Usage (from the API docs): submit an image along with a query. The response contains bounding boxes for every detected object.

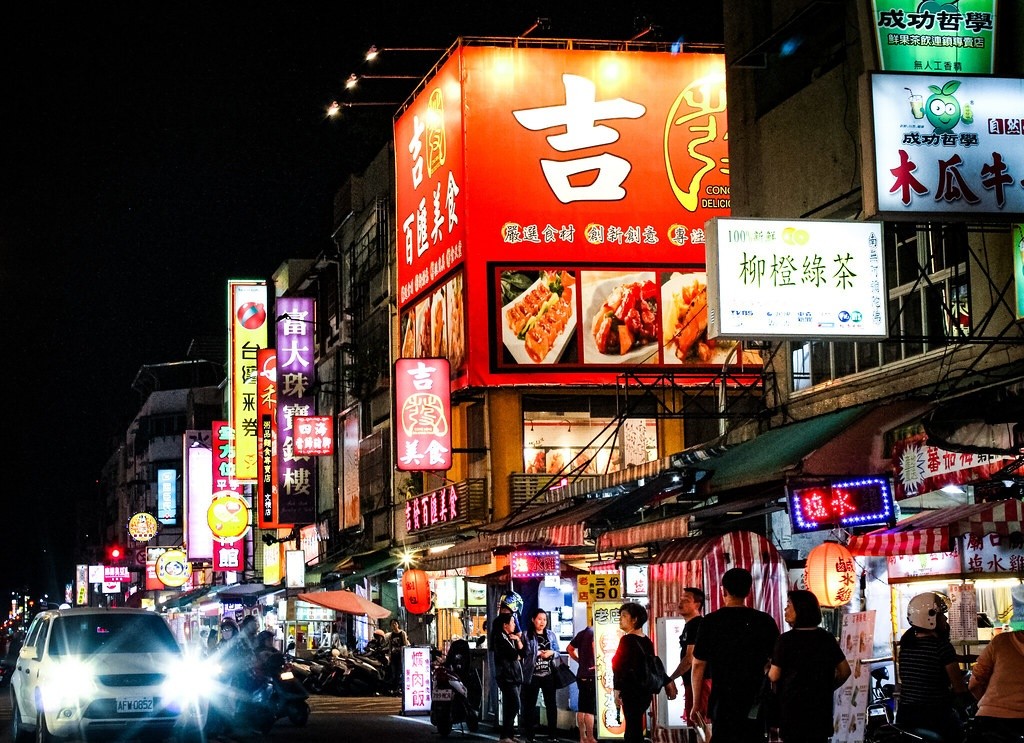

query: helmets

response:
[241,614,257,625]
[906,592,946,629]
[257,631,275,641]
[373,629,385,639]
[499,591,524,616]
[220,617,239,634]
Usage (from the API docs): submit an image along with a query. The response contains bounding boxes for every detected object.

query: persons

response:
[519,609,560,743]
[389,619,411,660]
[566,618,599,743]
[492,614,523,743]
[764,590,852,743]
[690,569,780,743]
[670,587,712,743]
[198,614,279,723]
[968,630,1024,743]
[612,602,678,743]
[896,592,964,732]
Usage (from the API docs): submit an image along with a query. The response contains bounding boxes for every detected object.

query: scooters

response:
[430,639,484,735]
[196,639,401,730]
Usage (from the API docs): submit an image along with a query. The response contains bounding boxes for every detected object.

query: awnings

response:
[156,587,211,611]
[321,547,385,573]
[296,590,391,620]
[597,491,787,555]
[848,499,1024,555]
[345,556,403,582]
[411,497,621,570]
[218,584,285,604]
[690,406,870,487]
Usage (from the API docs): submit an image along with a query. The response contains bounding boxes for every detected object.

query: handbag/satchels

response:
[550,653,576,689]
[618,634,665,694]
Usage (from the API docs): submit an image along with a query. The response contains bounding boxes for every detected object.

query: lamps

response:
[520,16,552,37]
[366,44,447,61]
[328,100,400,115]
[346,73,424,88]
[275,313,316,324]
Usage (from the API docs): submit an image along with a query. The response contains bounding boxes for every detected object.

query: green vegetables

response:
[501,270,563,302]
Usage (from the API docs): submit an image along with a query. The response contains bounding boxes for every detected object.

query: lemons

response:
[520,293,559,333]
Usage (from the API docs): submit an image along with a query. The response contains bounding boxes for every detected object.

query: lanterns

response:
[401,569,431,614]
[804,540,854,609]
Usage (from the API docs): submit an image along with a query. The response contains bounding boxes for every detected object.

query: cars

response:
[8,605,209,743]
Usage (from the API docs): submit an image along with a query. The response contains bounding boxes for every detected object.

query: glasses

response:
[221,629,234,632]
